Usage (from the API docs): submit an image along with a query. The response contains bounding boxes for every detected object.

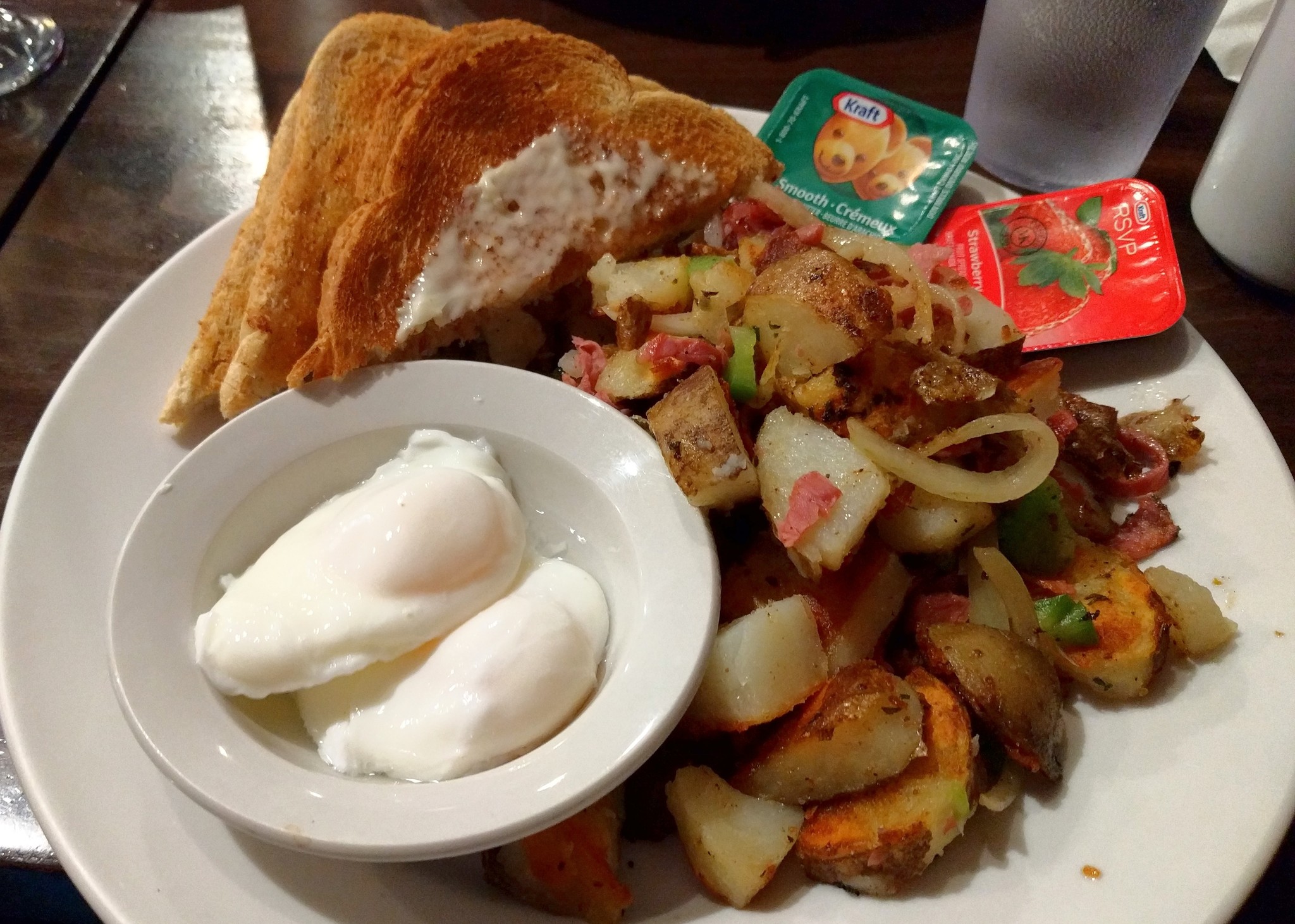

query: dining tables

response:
[3,5,1290,924]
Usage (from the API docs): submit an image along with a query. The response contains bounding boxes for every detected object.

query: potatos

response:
[536,200,1248,905]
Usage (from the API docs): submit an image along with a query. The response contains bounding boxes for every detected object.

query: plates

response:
[99,352,722,870]
[2,102,1293,924]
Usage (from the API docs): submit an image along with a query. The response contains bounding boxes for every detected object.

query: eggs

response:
[178,427,615,791]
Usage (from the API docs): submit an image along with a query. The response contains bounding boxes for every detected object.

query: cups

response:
[1186,0,1295,297]
[961,0,1231,214]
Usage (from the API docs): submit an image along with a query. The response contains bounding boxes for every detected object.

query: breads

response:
[146,13,794,466]
